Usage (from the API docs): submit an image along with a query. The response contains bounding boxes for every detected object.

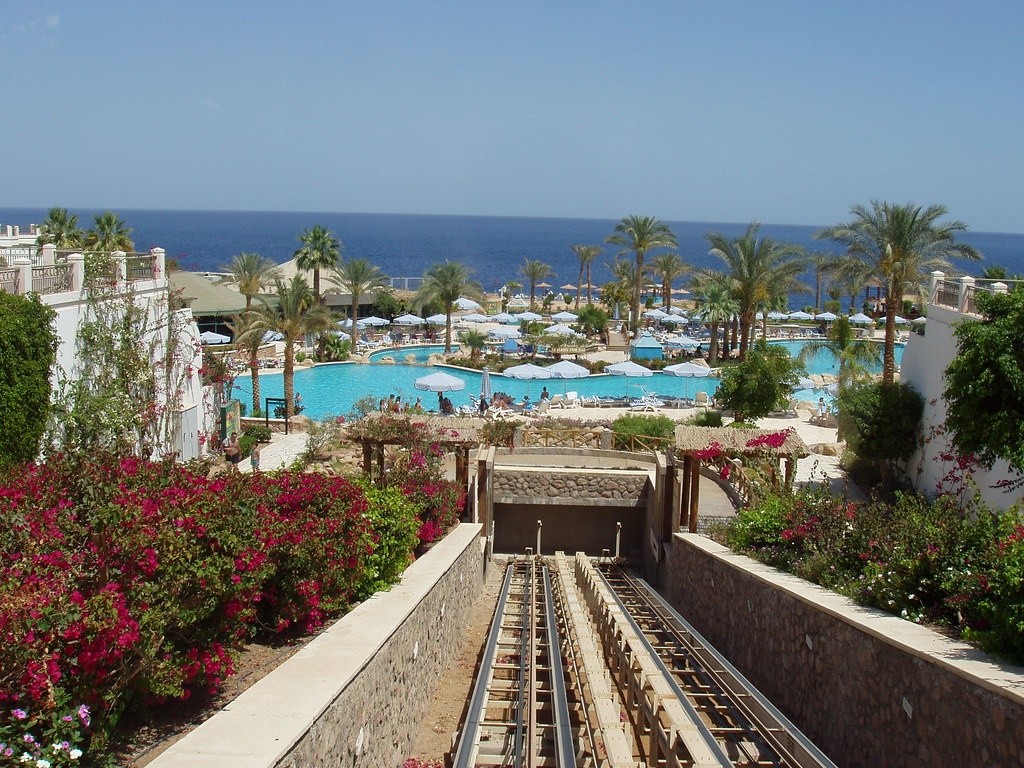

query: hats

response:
[543,387,546,390]
[417,397,422,402]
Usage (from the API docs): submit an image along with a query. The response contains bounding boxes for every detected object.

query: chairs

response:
[228,357,285,372]
[456,391,665,419]
[694,392,709,407]
[809,409,826,421]
[356,338,444,356]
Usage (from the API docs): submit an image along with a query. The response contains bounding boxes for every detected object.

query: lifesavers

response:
[517,346,526,356]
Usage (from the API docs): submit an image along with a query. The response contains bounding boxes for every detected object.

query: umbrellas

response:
[200,330,284,344]
[393,298,583,341]
[544,359,712,406]
[317,316,389,344]
[643,305,926,338]
[414,371,465,392]
[524,282,689,301]
[502,363,553,404]
[665,336,700,357]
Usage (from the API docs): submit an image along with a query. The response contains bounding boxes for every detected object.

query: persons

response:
[250,443,260,472]
[480,343,503,354]
[789,331,794,340]
[541,387,548,399]
[517,343,534,353]
[296,392,301,402]
[221,433,243,473]
[816,396,826,425]
[378,391,535,418]
[711,386,720,408]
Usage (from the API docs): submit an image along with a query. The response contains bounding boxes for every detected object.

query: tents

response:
[631,331,662,360]
